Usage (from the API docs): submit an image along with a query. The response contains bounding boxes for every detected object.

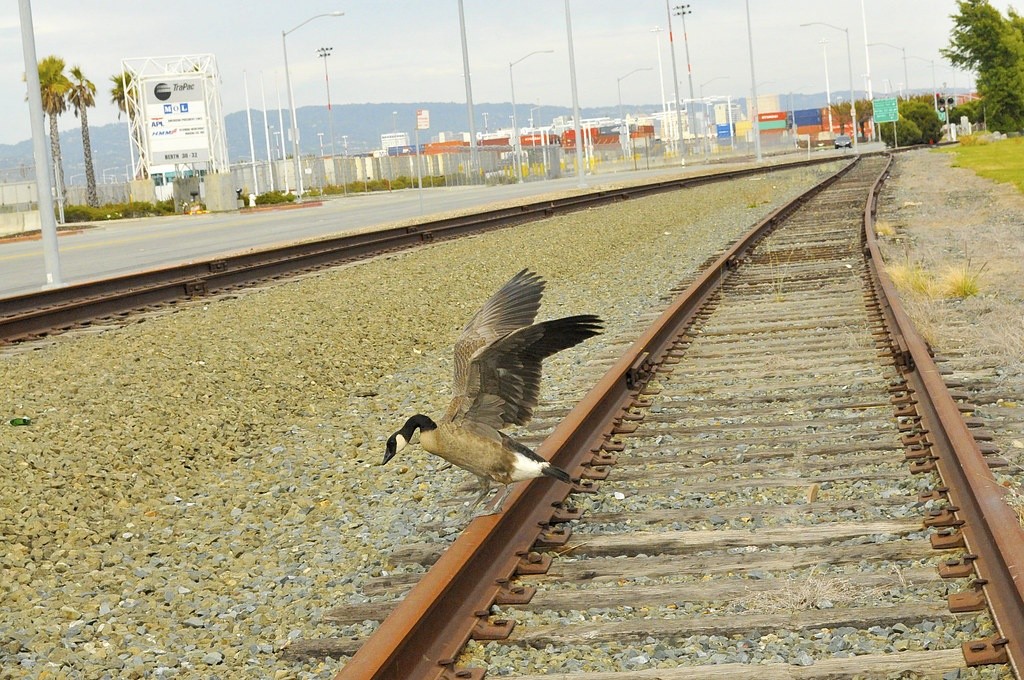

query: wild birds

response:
[382,269,605,482]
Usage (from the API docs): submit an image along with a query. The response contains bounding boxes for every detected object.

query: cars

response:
[833,135,852,149]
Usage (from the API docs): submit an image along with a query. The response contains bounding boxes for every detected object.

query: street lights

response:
[700,74,730,98]
[391,111,400,156]
[481,113,490,134]
[818,37,834,137]
[509,49,555,183]
[342,136,349,157]
[313,46,335,161]
[509,115,516,134]
[283,10,347,201]
[800,22,859,154]
[652,25,672,141]
[672,5,702,156]
[617,67,654,169]
[103,166,120,184]
[902,55,940,116]
[316,132,326,156]
[69,173,84,186]
[273,130,282,157]
[316,133,326,159]
[530,104,545,129]
[126,161,139,183]
[867,43,912,103]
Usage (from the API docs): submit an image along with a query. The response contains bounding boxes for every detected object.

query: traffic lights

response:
[939,98,945,104]
[948,98,954,104]
